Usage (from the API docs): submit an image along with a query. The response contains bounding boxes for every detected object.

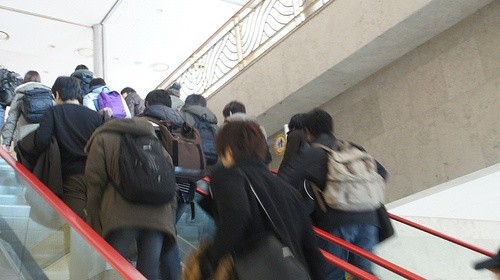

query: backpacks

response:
[76,75,94,103]
[310,139,387,212]
[20,87,54,122]
[98,86,126,118]
[108,131,175,206]
[142,116,206,183]
[193,116,219,164]
[0,69,24,106]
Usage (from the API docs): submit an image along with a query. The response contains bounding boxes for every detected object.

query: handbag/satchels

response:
[232,234,311,280]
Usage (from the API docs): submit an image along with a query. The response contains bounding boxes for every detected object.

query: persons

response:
[121,88,146,116]
[222,101,267,140]
[0,66,24,143]
[282,108,388,280]
[2,71,56,175]
[82,78,133,119]
[278,112,307,174]
[181,95,220,166]
[166,83,184,111]
[71,65,95,94]
[34,77,104,223]
[191,120,332,280]
[82,116,178,280]
[138,90,205,220]
